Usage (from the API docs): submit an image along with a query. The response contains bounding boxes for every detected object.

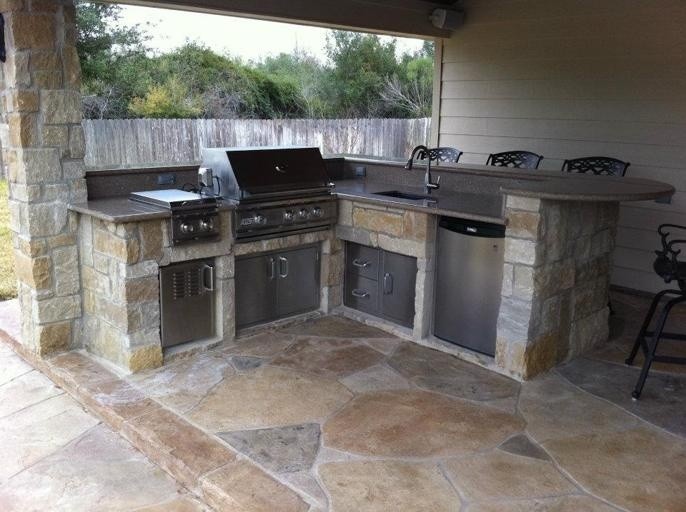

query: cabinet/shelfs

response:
[158,258,217,349]
[381,240,416,328]
[345,244,380,312]
[234,241,322,331]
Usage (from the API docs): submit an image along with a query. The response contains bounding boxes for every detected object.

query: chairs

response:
[485,151,544,170]
[417,146,463,166]
[561,156,631,177]
[629,223,685,398]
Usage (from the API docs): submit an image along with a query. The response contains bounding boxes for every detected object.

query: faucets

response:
[405,145,441,194]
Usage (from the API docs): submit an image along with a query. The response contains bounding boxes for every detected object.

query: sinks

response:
[371,190,434,201]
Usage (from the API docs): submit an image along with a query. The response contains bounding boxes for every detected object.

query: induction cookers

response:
[126,188,221,245]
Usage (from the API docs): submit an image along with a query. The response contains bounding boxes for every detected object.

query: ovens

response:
[433,216,506,357]
